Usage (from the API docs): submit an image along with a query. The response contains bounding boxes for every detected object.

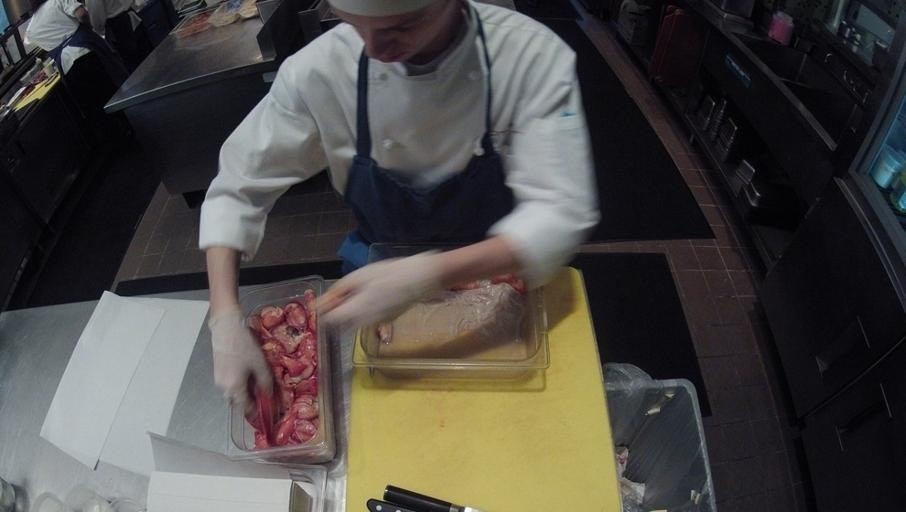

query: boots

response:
[117,127,143,157]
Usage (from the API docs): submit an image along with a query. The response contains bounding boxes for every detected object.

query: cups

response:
[42,63,54,77]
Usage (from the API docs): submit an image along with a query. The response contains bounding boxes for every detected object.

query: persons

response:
[85,0,158,73]
[198,1,601,432]
[22,0,130,136]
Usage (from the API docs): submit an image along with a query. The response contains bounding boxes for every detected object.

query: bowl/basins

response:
[19,63,38,84]
